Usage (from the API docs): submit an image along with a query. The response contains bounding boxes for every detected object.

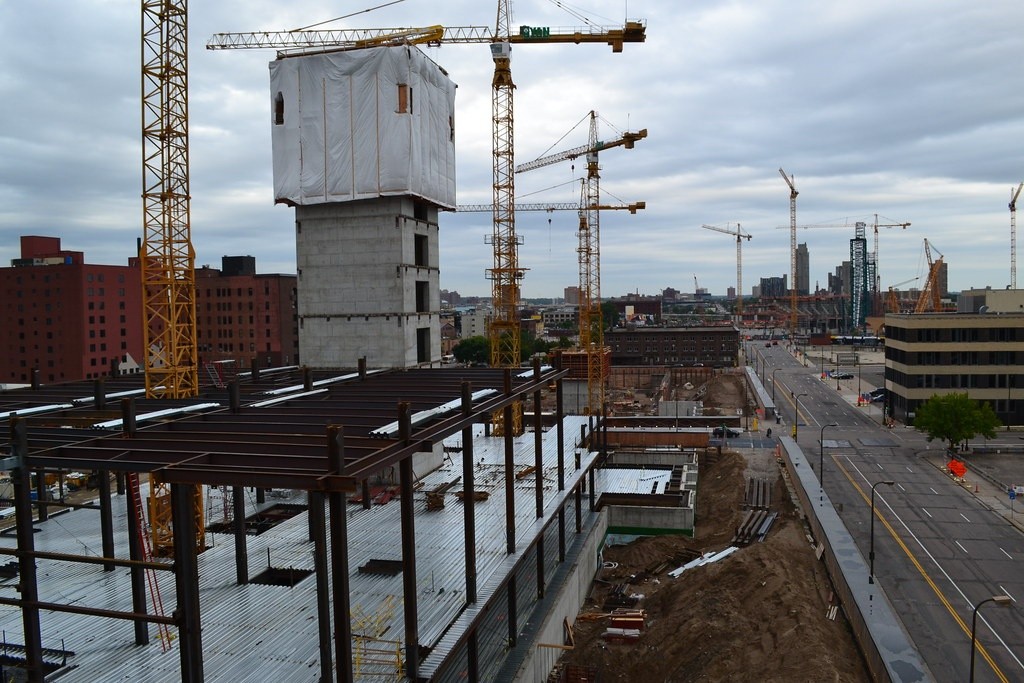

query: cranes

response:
[778,166,800,334]
[1007,182,1024,290]
[452,177,646,350]
[132,0,209,561]
[700,221,753,315]
[515,109,649,416]
[774,213,913,316]
[203,0,649,438]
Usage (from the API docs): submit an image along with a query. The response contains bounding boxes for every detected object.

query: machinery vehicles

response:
[887,238,945,314]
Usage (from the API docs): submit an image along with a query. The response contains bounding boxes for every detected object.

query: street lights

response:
[750,344,757,363]
[868,481,895,584]
[819,423,837,507]
[772,368,782,403]
[795,393,808,444]
[854,362,862,406]
[969,595,1010,683]
[963,387,969,451]
[762,355,772,387]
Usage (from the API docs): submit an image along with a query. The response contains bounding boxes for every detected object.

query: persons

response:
[766,428,771,438]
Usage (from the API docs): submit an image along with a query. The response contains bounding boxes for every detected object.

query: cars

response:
[712,427,739,439]
[830,371,885,402]
